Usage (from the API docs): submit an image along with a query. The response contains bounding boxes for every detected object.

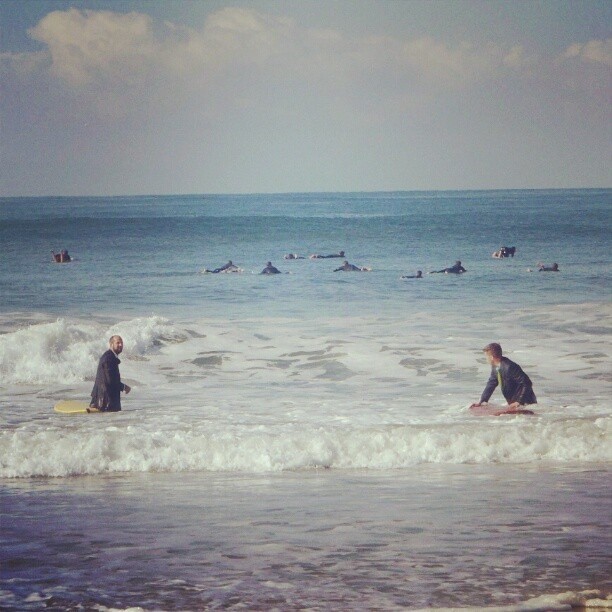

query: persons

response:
[334,260,372,272]
[90,334,132,413]
[202,259,236,273]
[51,247,72,263]
[492,246,518,259]
[468,342,539,410]
[284,253,306,259]
[400,270,423,280]
[526,261,560,273]
[309,250,346,259]
[257,261,294,275]
[426,260,468,274]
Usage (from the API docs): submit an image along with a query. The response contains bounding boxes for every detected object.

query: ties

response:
[496,367,502,388]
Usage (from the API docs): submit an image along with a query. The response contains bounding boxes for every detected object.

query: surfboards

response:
[54,399,99,413]
[470,403,534,416]
